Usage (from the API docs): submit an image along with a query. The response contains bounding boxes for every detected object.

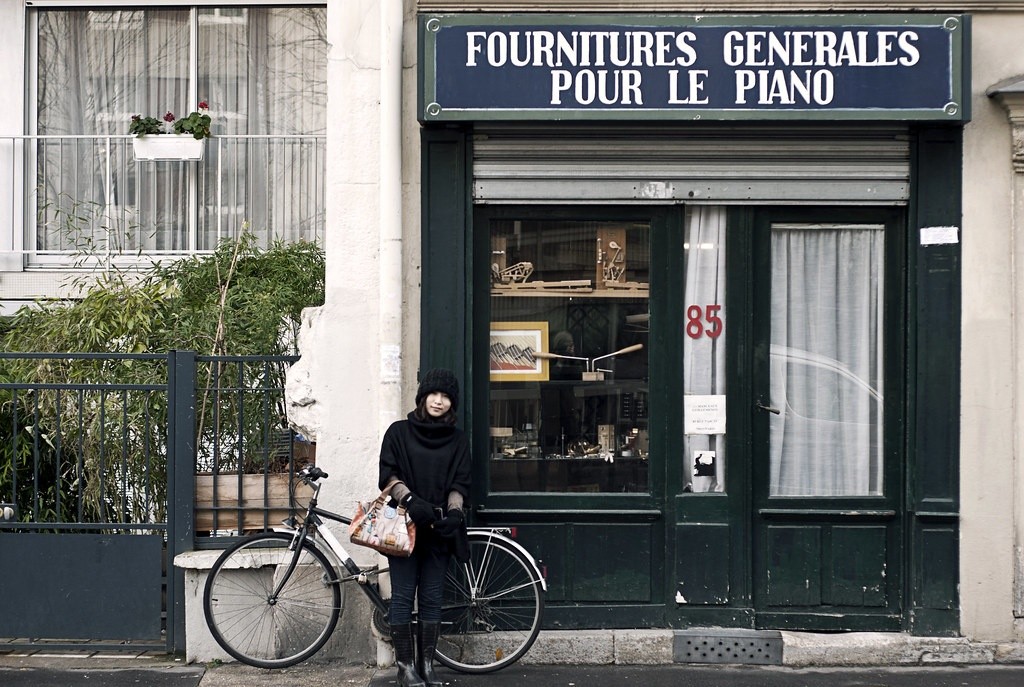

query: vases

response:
[132,133,206,162]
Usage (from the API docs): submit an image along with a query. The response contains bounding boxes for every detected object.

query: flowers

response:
[127,101,212,140]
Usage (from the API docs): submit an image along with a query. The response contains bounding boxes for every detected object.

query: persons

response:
[378,366,477,687]
[539,331,582,455]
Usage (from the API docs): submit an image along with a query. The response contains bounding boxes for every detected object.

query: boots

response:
[416,619,446,687]
[390,622,425,687]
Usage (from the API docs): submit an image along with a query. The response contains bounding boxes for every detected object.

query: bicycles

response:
[203,464,551,675]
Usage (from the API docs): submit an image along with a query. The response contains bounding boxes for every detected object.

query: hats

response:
[415,368,459,414]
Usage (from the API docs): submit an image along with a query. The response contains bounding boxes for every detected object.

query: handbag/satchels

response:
[348,479,417,557]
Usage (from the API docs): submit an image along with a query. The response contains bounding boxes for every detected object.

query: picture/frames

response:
[489,321,550,382]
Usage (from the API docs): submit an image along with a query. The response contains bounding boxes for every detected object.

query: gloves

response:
[401,491,436,531]
[447,509,464,547]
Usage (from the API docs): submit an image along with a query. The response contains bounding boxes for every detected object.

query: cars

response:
[768,344,883,496]
